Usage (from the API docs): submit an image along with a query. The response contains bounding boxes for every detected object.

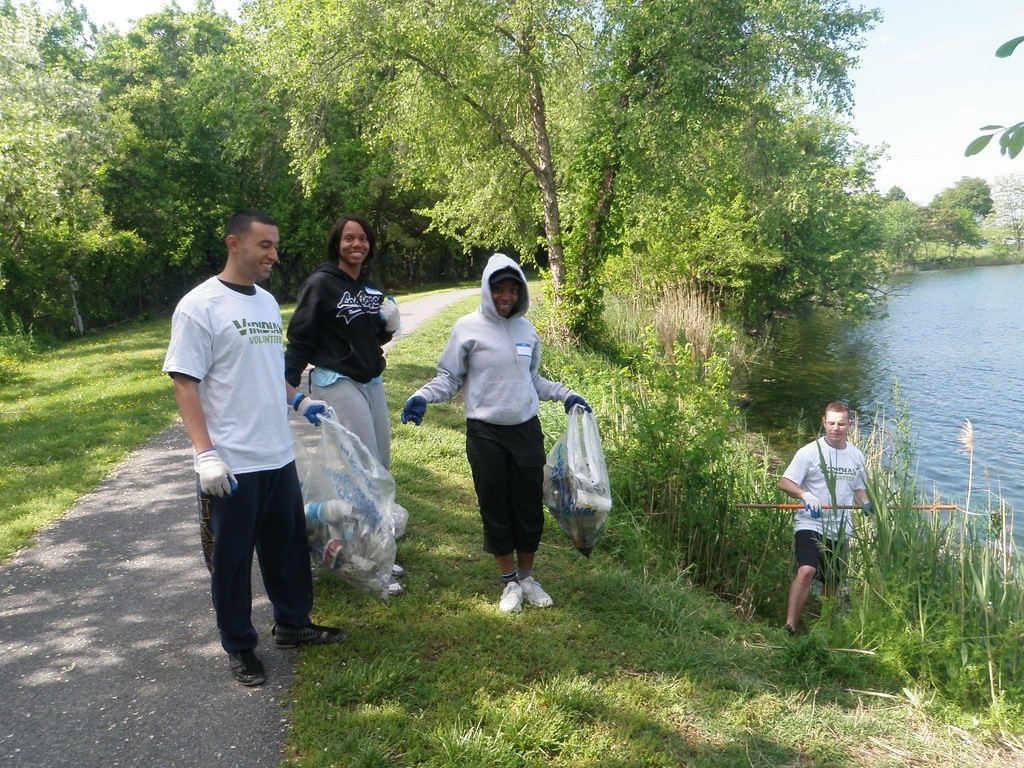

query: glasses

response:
[825,418,849,428]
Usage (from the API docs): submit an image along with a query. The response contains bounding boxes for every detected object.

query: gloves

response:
[197,449,238,497]
[801,492,822,519]
[378,295,400,331]
[401,391,427,426]
[565,395,592,415]
[291,392,330,426]
[861,501,873,517]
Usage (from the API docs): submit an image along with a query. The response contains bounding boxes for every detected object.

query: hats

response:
[489,268,525,284]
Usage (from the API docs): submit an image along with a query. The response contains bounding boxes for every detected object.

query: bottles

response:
[305,501,353,521]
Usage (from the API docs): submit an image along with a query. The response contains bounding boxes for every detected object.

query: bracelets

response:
[290,392,307,411]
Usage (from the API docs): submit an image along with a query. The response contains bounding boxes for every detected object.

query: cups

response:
[571,489,612,513]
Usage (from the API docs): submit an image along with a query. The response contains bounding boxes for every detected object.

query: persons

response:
[401,253,592,613]
[284,215,405,597]
[162,211,346,687]
[779,402,873,637]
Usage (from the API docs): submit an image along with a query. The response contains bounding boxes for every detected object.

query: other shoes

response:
[391,564,404,578]
[783,624,795,637]
[389,576,403,596]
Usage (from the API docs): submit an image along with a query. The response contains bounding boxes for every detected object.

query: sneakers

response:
[498,582,523,613]
[229,649,265,686]
[516,573,553,607]
[271,614,347,649]
